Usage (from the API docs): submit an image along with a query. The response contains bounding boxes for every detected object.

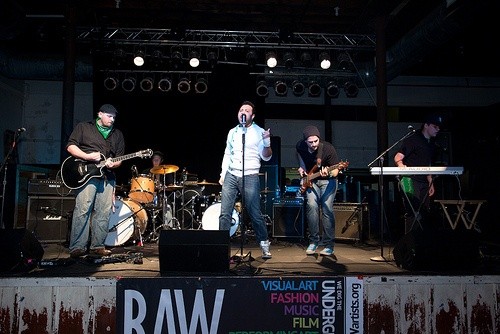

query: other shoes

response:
[70,249,86,258]
[89,248,112,255]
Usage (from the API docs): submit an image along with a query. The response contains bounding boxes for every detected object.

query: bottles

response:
[296,189,301,197]
[132,240,136,259]
[274,185,281,203]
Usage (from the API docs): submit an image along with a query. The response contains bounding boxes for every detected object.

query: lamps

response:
[272,77,289,97]
[338,53,352,70]
[89,40,219,69]
[245,48,257,67]
[341,77,359,98]
[324,78,340,98]
[255,76,270,97]
[300,53,314,72]
[265,51,279,69]
[290,79,305,97]
[100,69,213,94]
[319,51,332,70]
[282,52,297,70]
[308,79,322,97]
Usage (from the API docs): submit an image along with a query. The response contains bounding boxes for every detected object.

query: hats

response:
[303,125,320,142]
[99,104,117,114]
[425,113,444,126]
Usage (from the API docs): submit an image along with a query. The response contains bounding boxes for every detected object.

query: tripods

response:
[143,173,181,244]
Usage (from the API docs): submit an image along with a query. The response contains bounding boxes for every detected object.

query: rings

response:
[263,133,265,134]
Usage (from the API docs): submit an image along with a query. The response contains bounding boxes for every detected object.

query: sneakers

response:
[306,243,318,255]
[260,240,272,257]
[320,248,334,255]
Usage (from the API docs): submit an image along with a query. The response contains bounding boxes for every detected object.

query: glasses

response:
[306,138,319,144]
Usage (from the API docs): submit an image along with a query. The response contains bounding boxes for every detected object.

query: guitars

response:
[299,159,350,196]
[60,148,153,190]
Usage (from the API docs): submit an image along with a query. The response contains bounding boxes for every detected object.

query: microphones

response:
[242,114,246,129]
[407,123,416,132]
[15,128,26,132]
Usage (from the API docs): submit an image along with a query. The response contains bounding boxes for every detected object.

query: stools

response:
[432,198,488,258]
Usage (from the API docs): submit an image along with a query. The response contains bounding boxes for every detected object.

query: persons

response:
[143,151,174,228]
[66,104,125,255]
[218,101,272,258]
[295,126,339,255]
[394,114,441,234]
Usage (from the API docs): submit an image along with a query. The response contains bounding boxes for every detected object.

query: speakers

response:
[394,231,480,276]
[23,196,76,243]
[272,205,305,237]
[0,230,44,271]
[159,229,230,276]
[318,205,362,240]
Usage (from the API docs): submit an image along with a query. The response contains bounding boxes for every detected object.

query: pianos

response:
[369,166,465,261]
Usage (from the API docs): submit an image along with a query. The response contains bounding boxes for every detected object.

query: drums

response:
[172,189,241,238]
[102,174,165,246]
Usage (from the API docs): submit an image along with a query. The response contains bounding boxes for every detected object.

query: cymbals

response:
[260,190,279,193]
[150,164,179,175]
[196,182,220,186]
[165,184,184,190]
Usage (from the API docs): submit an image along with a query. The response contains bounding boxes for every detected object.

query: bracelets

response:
[328,172,331,177]
[396,160,402,164]
